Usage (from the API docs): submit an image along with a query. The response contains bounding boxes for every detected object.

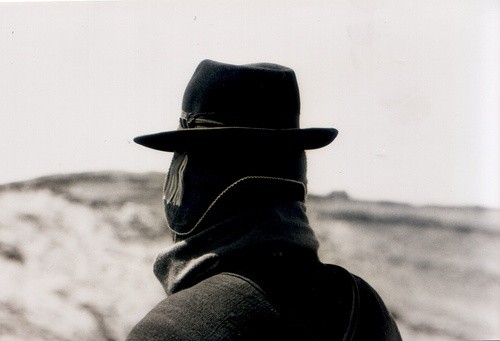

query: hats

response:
[132,59,338,153]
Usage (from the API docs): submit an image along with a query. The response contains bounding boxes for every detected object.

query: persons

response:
[127,60,402,341]
